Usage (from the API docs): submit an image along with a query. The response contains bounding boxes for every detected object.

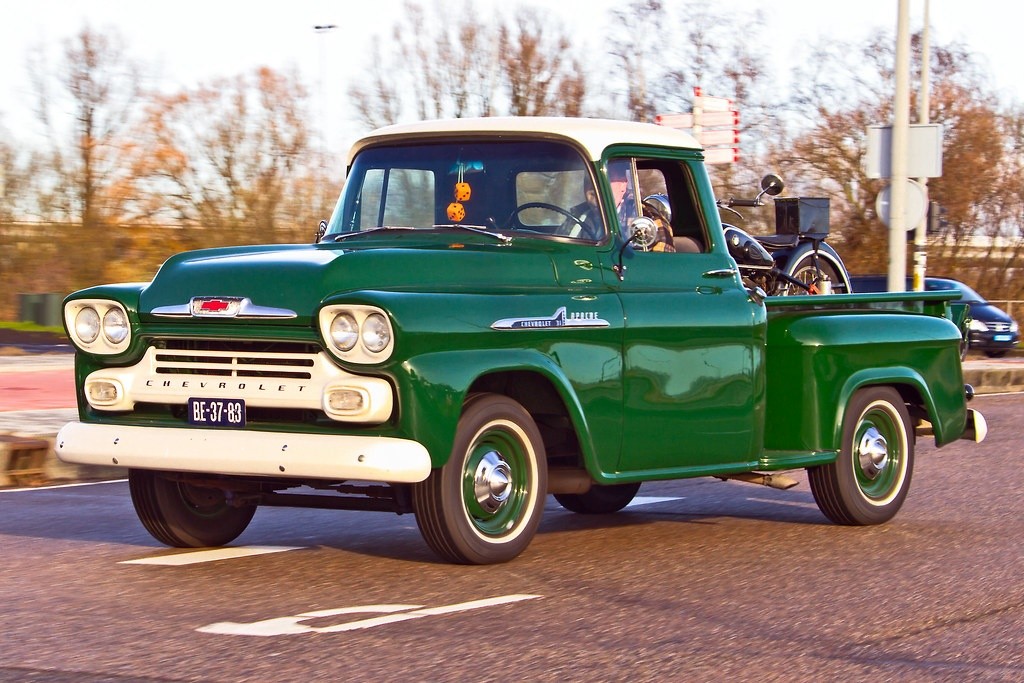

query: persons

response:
[551,159,677,253]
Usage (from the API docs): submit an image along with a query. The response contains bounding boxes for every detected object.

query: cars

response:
[848,273,1022,358]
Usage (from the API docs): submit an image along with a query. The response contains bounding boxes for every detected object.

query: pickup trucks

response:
[54,114,988,566]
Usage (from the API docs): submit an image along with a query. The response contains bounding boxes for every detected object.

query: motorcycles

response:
[640,172,853,296]
